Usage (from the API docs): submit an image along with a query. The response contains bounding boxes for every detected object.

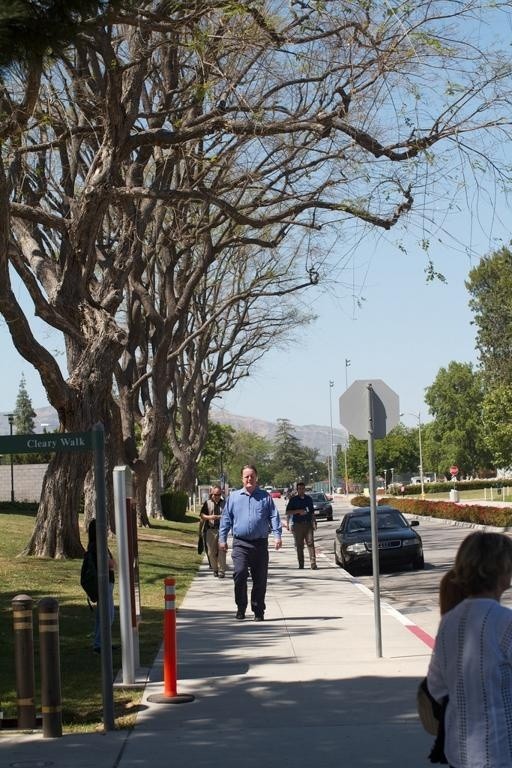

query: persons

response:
[80,517,115,656]
[286,483,317,570]
[198,486,225,578]
[416,533,512,768]
[220,465,282,621]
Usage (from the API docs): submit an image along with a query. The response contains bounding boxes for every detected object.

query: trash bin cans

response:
[497,488,501,495]
[449,489,459,502]
[364,488,370,496]
[337,488,342,494]
[321,488,324,493]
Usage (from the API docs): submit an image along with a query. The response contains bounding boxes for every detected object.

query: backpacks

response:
[80,548,98,604]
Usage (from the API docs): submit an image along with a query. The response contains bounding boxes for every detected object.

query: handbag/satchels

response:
[198,536,204,555]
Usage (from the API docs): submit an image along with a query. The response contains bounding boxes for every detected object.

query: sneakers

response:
[214,570,225,578]
[299,561,318,570]
[235,607,264,622]
[92,643,122,654]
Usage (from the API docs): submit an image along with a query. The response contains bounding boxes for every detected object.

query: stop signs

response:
[450,466,459,475]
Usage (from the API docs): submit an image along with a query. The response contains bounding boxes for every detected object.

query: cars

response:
[265,487,280,499]
[305,492,332,521]
[333,505,423,576]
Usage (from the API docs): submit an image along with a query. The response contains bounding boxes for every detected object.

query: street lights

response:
[345,358,351,493]
[3,411,17,501]
[218,450,224,477]
[400,410,425,495]
[389,468,395,484]
[383,469,387,491]
[329,377,335,491]
